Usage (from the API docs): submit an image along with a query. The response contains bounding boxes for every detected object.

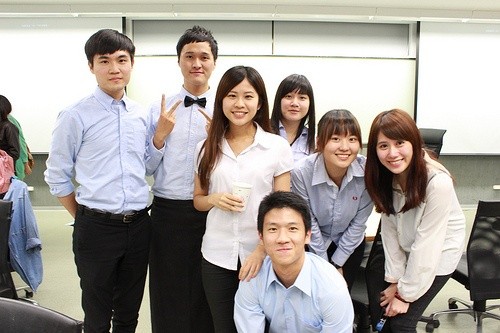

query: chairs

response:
[0,298,83,333]
[419,128,447,159]
[430,198,500,332]
[0,199,39,309]
[2,178,43,297]
[348,214,436,333]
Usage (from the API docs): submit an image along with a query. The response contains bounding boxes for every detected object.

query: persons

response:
[365,110,466,333]
[43,29,148,333]
[144,24,218,333]
[269,72,316,164]
[294,109,376,295]
[193,65,296,333]
[233,190,356,333]
[0,95,33,181]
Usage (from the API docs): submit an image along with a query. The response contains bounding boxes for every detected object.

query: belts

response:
[77,205,148,222]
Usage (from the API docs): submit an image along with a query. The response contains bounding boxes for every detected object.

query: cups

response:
[231,184,254,213]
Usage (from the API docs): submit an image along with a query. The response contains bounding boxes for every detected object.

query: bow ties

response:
[184,95,206,107]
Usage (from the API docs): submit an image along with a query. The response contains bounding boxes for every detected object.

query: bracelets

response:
[395,295,410,303]
